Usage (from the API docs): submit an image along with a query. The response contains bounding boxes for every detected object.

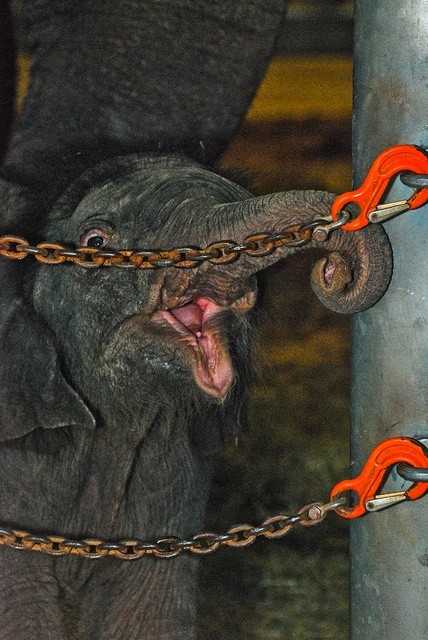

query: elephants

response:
[0,123,398,640]
